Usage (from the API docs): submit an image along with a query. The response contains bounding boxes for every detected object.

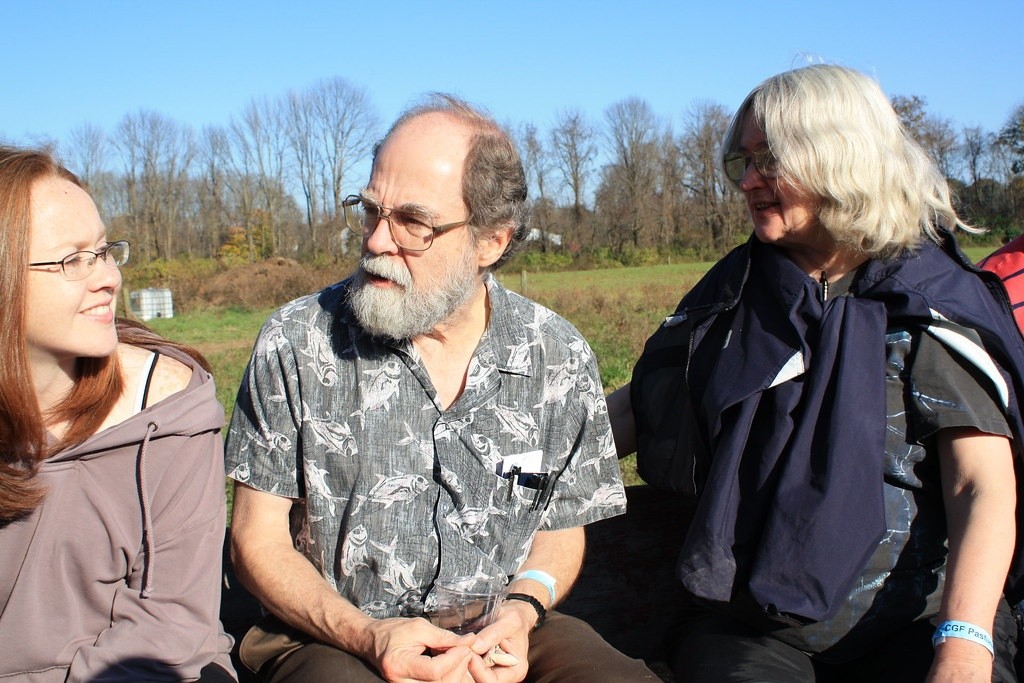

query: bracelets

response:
[933,621,998,659]
[510,569,556,603]
[507,593,545,630]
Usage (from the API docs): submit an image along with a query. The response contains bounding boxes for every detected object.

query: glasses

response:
[29,239,131,281]
[723,152,789,182]
[343,195,475,251]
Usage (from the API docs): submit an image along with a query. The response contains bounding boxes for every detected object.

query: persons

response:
[0,144,237,682]
[606,65,1023,683]
[224,95,663,683]
